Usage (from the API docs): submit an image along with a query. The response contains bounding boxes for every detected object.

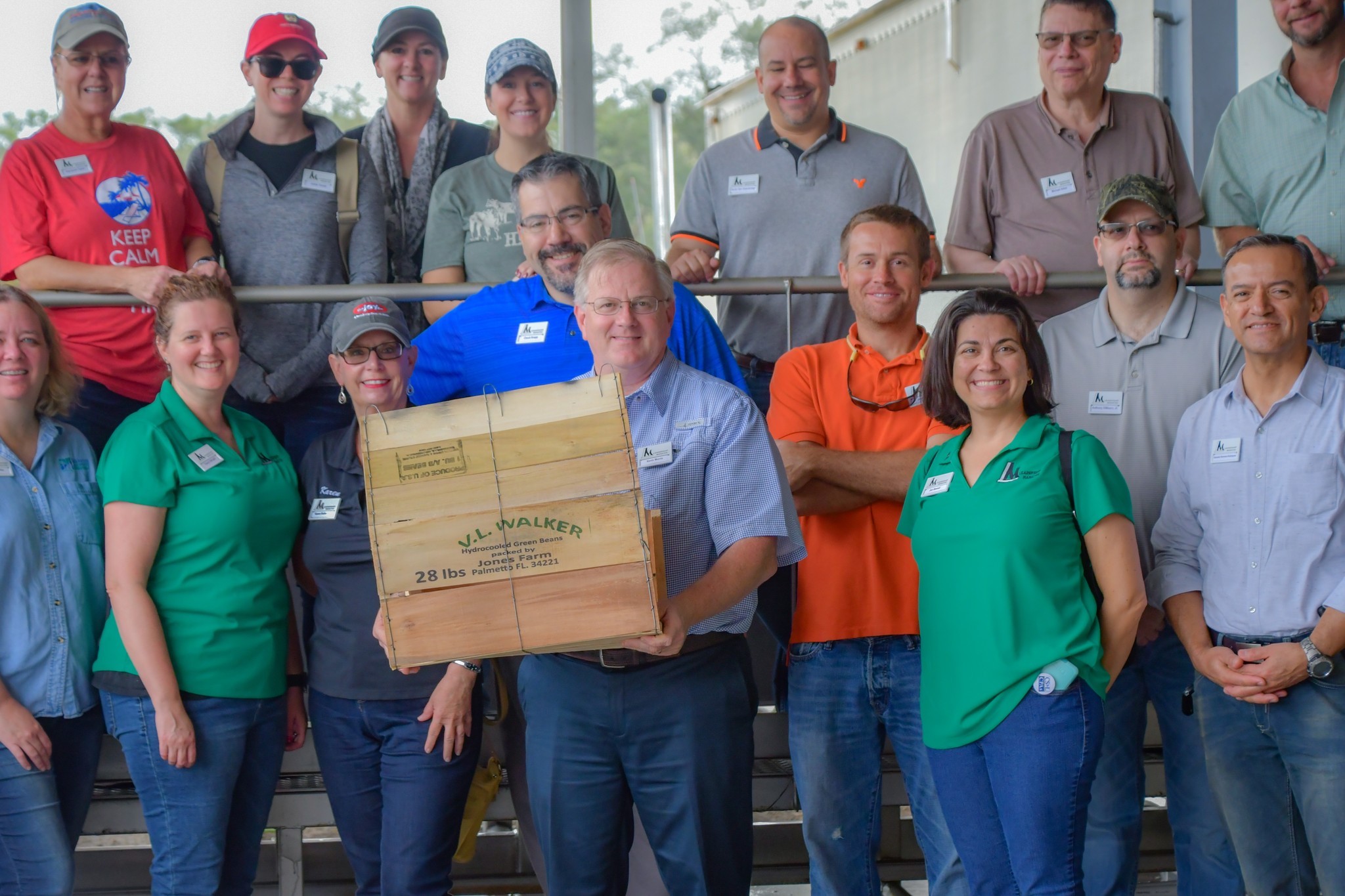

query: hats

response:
[371,6,449,63]
[485,38,556,86]
[1096,172,1175,223]
[244,12,327,60]
[51,3,129,56]
[331,296,412,355]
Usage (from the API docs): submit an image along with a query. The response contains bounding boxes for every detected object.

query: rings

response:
[1172,268,1187,274]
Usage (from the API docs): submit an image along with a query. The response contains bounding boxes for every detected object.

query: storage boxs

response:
[354,373,668,670]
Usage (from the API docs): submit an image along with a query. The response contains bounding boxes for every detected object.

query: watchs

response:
[452,660,482,674]
[1299,638,1335,679]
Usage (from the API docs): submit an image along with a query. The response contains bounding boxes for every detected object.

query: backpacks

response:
[452,751,504,864]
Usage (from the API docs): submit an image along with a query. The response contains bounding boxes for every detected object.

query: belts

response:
[728,346,777,375]
[1303,319,1345,346]
[557,631,742,670]
[1204,629,1278,664]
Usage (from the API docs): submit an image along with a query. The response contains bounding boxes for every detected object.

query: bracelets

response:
[189,255,220,267]
[283,671,309,691]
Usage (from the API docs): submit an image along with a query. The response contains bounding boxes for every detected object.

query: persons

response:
[420,34,643,329]
[1196,0,1344,368]
[1150,234,1345,895]
[2,2,237,460]
[941,0,1209,336]
[373,236,810,895]
[97,272,310,896]
[291,294,490,895]
[403,150,755,894]
[663,15,943,719]
[187,12,387,670]
[1037,173,1246,895]
[1,281,117,896]
[343,6,506,333]
[895,284,1149,896]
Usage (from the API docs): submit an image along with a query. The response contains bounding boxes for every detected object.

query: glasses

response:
[1036,28,1114,49]
[519,205,598,231]
[248,57,317,80]
[337,342,406,364]
[52,51,128,66]
[1098,219,1177,239]
[846,358,923,411]
[583,296,670,315]
